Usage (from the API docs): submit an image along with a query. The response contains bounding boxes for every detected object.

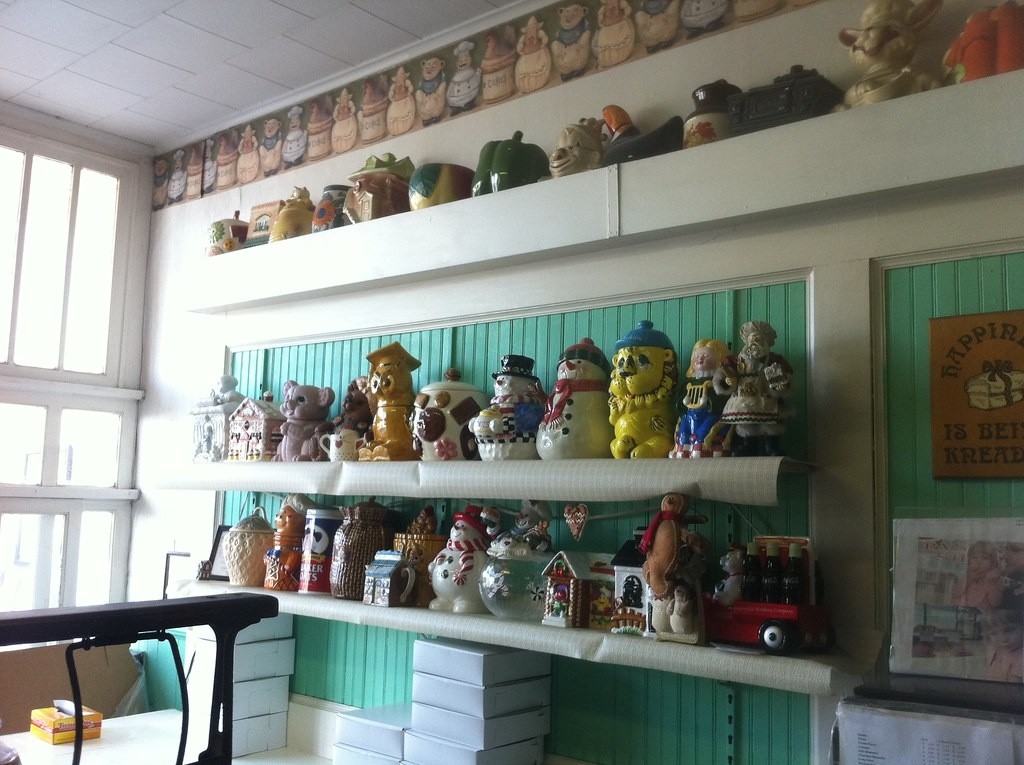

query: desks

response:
[0,711,332,765]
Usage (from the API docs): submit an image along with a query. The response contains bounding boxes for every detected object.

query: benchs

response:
[0,642,151,735]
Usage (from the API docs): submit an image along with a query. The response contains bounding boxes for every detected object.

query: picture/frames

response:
[207,524,229,581]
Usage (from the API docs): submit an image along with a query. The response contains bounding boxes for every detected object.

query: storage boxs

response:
[332,628,552,765]
[183,613,295,759]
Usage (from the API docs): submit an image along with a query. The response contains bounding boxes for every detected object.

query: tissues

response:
[30,699,102,746]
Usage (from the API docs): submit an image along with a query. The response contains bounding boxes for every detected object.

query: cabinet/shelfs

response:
[158,455,858,699]
